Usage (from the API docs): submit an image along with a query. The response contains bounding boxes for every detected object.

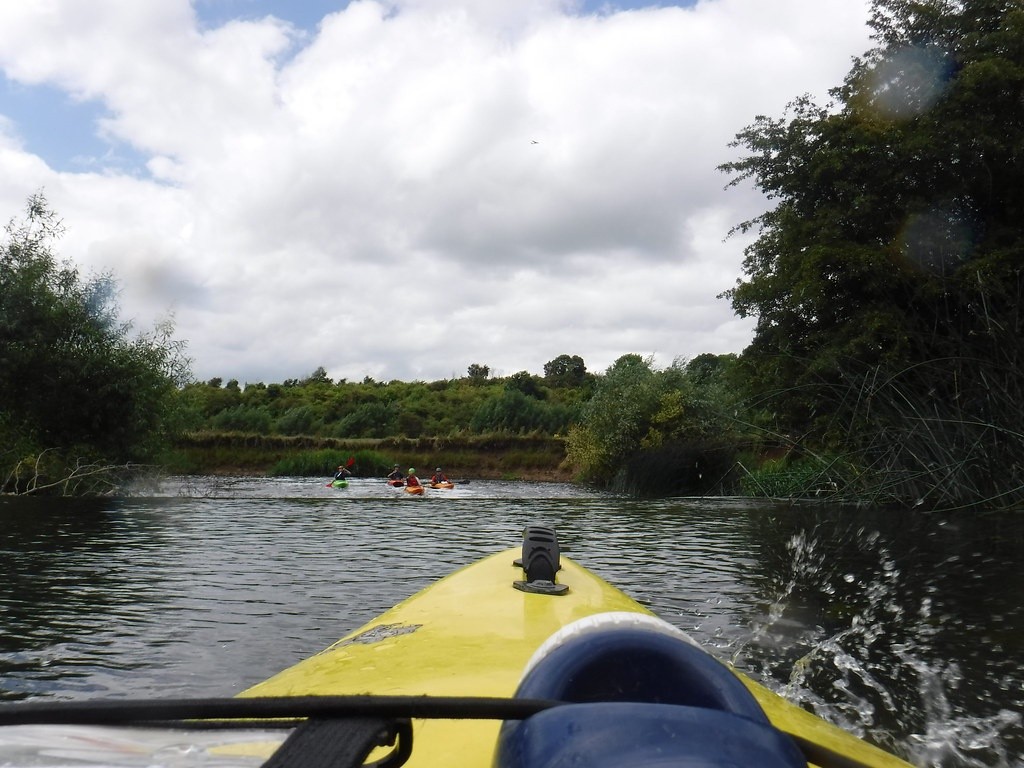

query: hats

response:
[408,468,415,475]
[394,464,400,466]
[337,466,343,469]
[436,468,441,471]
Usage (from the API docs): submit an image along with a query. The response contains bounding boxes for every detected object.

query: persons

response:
[407,468,421,487]
[335,466,351,480]
[386,464,406,481]
[431,467,447,485]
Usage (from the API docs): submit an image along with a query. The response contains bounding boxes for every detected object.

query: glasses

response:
[436,471,440,472]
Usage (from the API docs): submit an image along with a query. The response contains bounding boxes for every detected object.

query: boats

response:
[430,482,455,489]
[403,486,425,495]
[388,480,404,487]
[332,480,348,488]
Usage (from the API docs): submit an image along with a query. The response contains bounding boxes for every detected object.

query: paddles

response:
[421,479,470,485]
[326,457,354,488]
